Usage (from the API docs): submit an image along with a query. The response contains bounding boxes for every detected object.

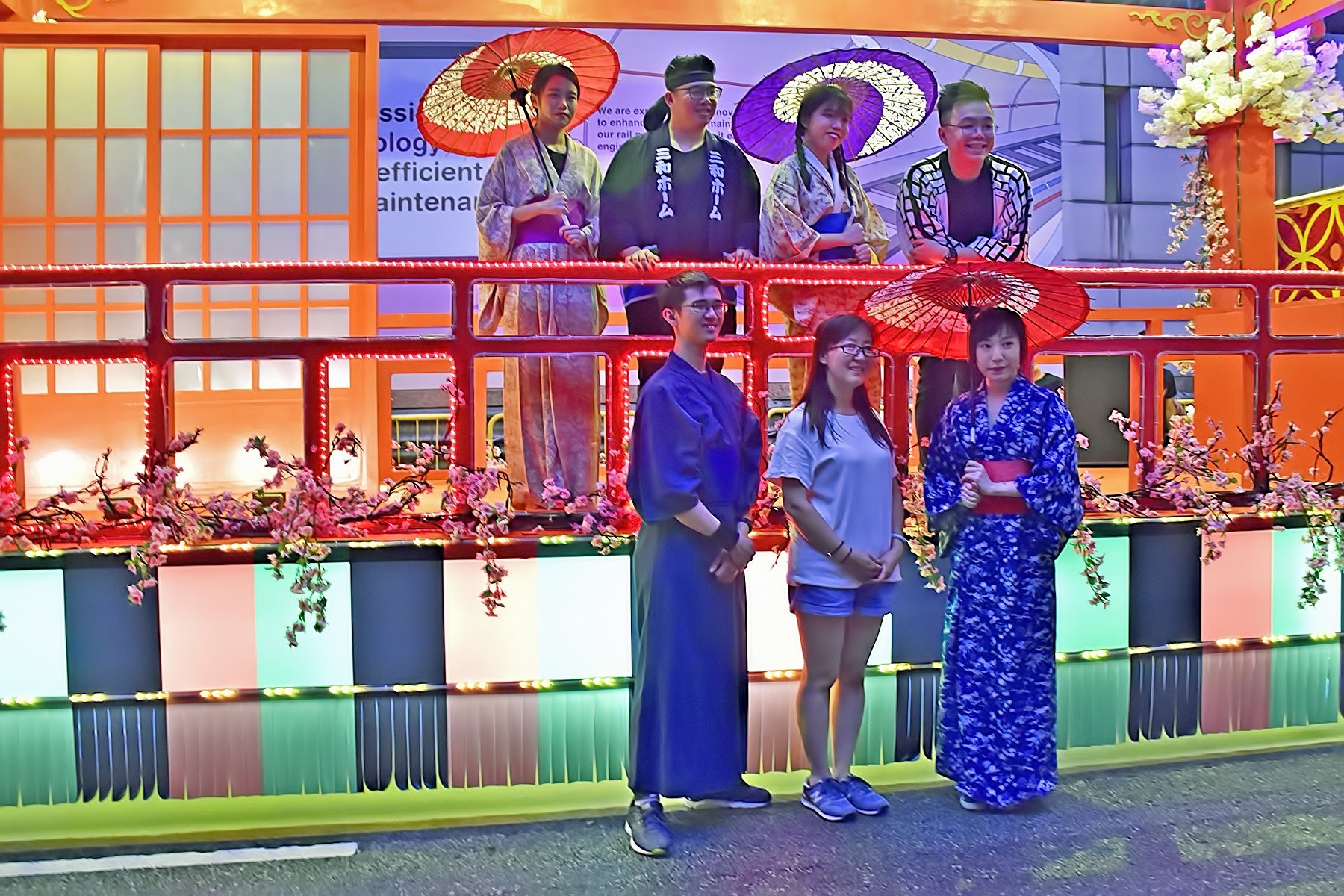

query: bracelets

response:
[826,540,845,557]
[891,533,907,543]
[736,518,753,533]
[840,547,852,565]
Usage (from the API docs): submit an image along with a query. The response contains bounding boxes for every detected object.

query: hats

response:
[663,53,716,90]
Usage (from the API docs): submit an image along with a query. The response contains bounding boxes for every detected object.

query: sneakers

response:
[801,777,856,820]
[624,800,674,855]
[833,773,890,815]
[683,775,772,809]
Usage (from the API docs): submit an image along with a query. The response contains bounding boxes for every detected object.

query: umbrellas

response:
[414,24,620,229]
[731,47,939,221]
[856,260,1097,459]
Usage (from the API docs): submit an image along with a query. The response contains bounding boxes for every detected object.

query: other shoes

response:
[959,791,986,810]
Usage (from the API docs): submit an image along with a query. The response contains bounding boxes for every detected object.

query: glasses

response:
[941,123,999,136]
[673,84,723,102]
[825,343,881,359]
[683,299,728,316]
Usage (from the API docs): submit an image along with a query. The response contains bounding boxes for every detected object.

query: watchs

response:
[943,245,957,264]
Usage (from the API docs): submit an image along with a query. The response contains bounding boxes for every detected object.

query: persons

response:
[597,53,761,397]
[923,307,1083,810]
[766,315,910,822]
[476,64,603,513]
[896,80,1035,475]
[761,85,889,408]
[623,272,772,856]
